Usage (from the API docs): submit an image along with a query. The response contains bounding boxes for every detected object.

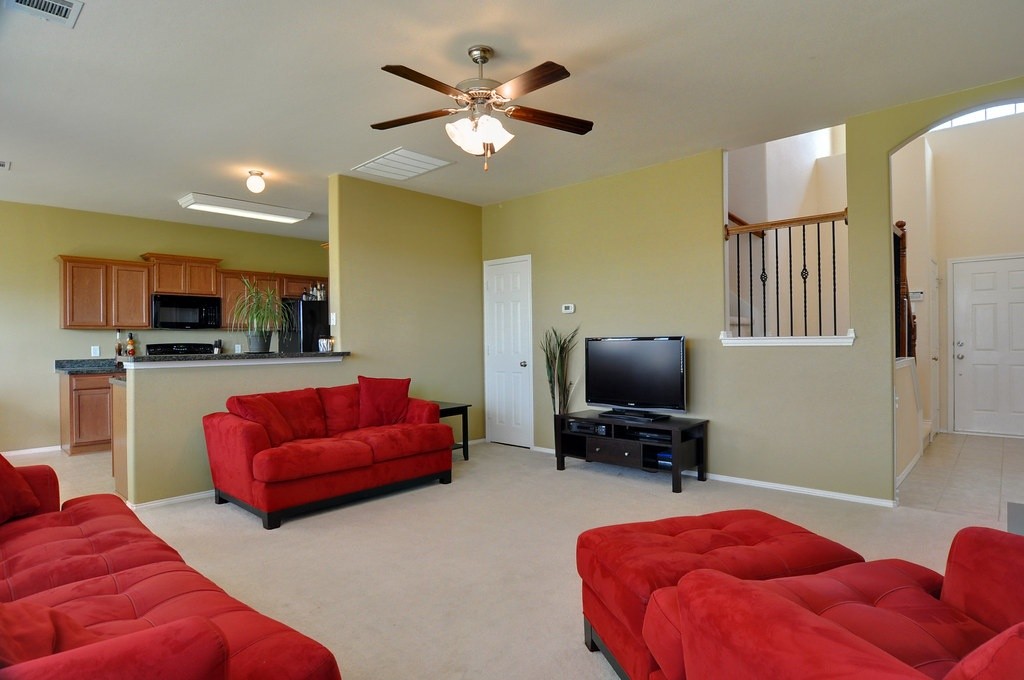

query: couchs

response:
[576,508,1024,680]
[202,375,453,530]
[0,452,341,680]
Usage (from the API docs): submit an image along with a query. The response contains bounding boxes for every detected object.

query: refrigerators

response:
[279,300,330,353]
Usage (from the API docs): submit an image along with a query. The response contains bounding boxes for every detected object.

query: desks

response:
[433,399,473,461]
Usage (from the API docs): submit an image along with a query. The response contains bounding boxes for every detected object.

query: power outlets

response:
[331,313,336,325]
[235,345,241,353]
[91,346,100,357]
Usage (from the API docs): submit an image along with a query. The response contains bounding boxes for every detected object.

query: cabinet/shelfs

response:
[59,374,129,501]
[554,410,710,493]
[140,251,224,297]
[54,255,154,330]
[282,274,328,299]
[219,269,281,333]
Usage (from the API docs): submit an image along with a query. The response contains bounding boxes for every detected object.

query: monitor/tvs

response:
[585,336,686,423]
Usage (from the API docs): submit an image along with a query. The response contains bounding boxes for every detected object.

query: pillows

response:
[357,375,411,429]
[0,453,40,525]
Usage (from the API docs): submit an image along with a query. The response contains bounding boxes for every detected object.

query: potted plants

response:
[225,273,298,353]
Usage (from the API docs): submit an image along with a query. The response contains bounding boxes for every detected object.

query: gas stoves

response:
[146,344,213,355]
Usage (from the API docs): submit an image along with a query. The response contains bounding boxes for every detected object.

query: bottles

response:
[124,332,135,357]
[114,328,123,356]
[302,282,326,301]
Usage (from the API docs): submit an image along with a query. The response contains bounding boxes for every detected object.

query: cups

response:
[214,347,221,355]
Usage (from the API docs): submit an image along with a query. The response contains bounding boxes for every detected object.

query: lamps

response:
[445,105,515,172]
[177,193,312,225]
[247,169,265,194]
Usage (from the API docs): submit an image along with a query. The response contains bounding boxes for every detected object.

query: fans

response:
[370,45,594,137]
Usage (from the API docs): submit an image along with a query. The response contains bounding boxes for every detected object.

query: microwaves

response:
[150,293,221,330]
